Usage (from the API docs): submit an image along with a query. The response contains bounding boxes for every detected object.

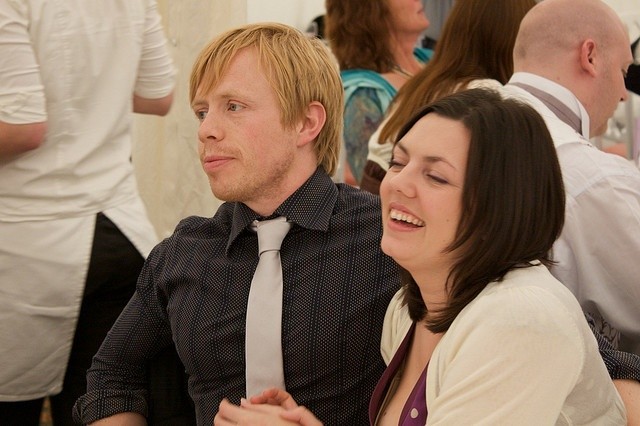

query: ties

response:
[245,214,289,405]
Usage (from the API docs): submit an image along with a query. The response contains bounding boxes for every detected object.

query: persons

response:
[360,0,539,195]
[214,91,627,426]
[0,0,176,426]
[307,1,436,189]
[492,0,640,354]
[73,21,409,426]
